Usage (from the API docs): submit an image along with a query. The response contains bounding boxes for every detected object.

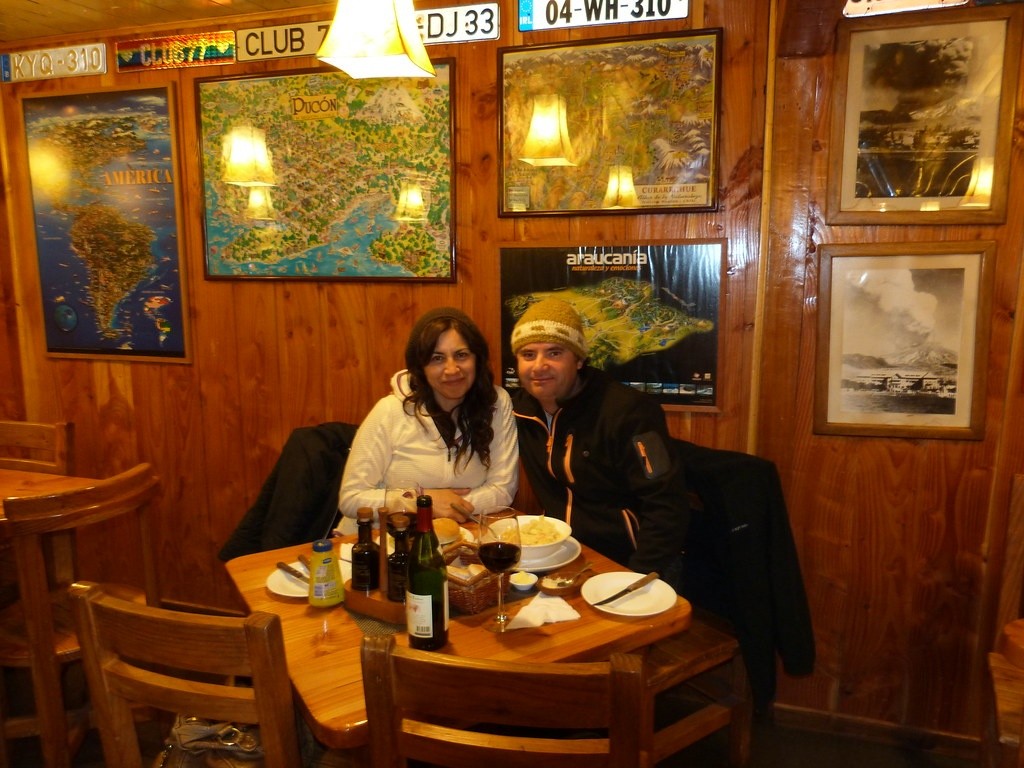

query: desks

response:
[226,507,694,768]
[1,469,105,713]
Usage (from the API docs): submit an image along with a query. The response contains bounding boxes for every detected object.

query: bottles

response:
[404,496,450,651]
[387,516,411,602]
[351,507,380,591]
[375,507,390,592]
[308,539,344,608]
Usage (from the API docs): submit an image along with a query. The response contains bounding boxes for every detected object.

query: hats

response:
[405,310,484,347]
[510,298,587,362]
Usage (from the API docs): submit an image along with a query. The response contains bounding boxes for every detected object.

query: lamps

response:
[960,156,996,207]
[317,0,437,79]
[221,93,642,223]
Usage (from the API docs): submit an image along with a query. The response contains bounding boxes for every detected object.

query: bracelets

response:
[416,487,424,496]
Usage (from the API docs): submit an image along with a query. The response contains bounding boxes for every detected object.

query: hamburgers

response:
[431,518,465,550]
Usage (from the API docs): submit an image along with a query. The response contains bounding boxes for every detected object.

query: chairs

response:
[0,420,779,766]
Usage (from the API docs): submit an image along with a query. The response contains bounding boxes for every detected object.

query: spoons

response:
[557,568,592,586]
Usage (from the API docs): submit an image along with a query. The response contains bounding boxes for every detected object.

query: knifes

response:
[591,572,659,606]
[277,561,309,584]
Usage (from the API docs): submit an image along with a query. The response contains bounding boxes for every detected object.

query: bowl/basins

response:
[537,572,585,595]
[486,515,572,561]
[510,573,538,591]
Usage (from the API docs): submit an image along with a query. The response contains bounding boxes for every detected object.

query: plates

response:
[375,527,475,555]
[339,543,395,561]
[511,536,581,572]
[265,559,352,597]
[580,572,677,617]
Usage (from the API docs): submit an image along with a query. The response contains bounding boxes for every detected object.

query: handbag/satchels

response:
[161,713,266,768]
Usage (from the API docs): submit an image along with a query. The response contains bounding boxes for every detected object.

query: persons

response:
[507,299,692,594]
[336,307,520,537]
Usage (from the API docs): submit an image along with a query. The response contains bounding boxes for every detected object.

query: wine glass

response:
[478,505,521,633]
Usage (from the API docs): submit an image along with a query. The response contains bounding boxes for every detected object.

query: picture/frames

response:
[496,24,726,220]
[193,56,457,285]
[16,80,192,365]
[496,236,726,416]
[822,3,1024,228]
[811,238,997,442]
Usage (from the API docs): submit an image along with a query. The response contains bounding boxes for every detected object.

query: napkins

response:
[505,589,581,632]
